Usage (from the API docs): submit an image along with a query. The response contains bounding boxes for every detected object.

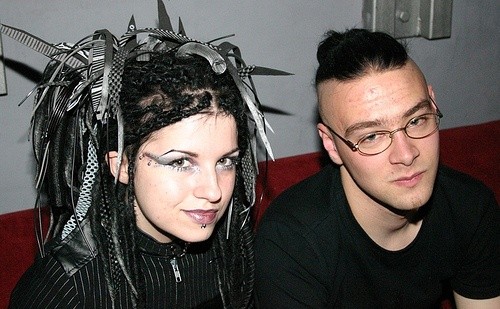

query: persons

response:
[255,26,500,309]
[0,0,296,309]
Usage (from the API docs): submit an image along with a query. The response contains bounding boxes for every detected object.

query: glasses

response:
[322,95,444,156]
